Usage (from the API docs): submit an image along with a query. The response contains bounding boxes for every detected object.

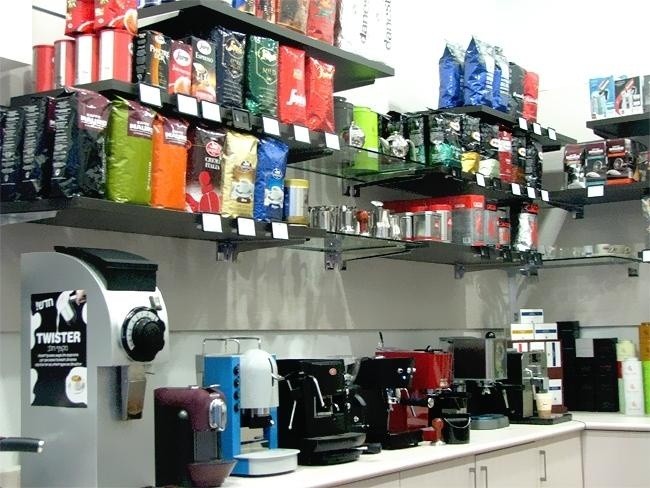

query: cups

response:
[311,205,338,234]
[612,244,631,258]
[596,243,615,255]
[337,205,357,234]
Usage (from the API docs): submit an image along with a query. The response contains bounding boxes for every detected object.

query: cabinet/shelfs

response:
[476,430,583,488]
[338,456,477,488]
[1,0,650,278]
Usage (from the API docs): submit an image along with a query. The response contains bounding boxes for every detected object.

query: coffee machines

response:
[375,345,471,445]
[346,356,435,450]
[439,333,525,430]
[19,248,170,487]
[276,359,368,464]
[507,349,573,425]
[194,336,300,475]
[155,384,238,487]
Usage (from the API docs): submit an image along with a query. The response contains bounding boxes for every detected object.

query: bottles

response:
[334,96,353,167]
[284,179,312,225]
[620,357,644,415]
[616,340,636,411]
[352,107,378,172]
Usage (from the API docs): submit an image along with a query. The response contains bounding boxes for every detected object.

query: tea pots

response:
[339,120,366,151]
[377,131,417,162]
